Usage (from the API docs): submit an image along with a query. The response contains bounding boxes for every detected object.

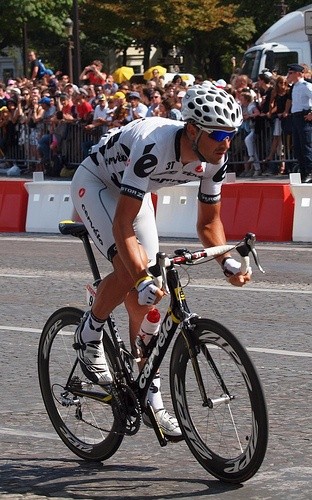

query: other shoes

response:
[240,169,275,177]
[302,175,312,183]
[248,157,256,162]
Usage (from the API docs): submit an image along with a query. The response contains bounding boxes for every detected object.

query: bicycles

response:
[35,219,271,487]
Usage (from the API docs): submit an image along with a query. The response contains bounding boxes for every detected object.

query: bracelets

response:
[135,276,152,288]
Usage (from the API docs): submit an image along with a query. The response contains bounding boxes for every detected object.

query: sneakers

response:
[73,324,113,385]
[143,409,182,436]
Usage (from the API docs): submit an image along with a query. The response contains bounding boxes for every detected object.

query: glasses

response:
[21,93,29,96]
[279,82,287,84]
[289,71,296,75]
[149,96,159,99]
[195,123,239,141]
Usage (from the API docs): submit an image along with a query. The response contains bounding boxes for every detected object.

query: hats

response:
[38,97,50,104]
[128,93,141,100]
[114,92,125,100]
[63,83,73,90]
[75,88,88,97]
[41,69,53,75]
[288,64,305,72]
[177,91,186,97]
[97,93,106,101]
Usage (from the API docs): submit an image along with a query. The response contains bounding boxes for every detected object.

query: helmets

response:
[182,84,243,127]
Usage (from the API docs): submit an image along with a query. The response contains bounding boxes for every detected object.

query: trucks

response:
[232,3,312,88]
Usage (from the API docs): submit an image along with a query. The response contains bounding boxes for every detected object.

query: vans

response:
[127,70,195,88]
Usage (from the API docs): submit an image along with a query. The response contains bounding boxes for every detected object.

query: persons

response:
[27,50,45,78]
[80,60,106,85]
[71,83,253,440]
[0,64,312,184]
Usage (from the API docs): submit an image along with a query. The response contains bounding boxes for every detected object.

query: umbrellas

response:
[112,66,135,84]
[143,66,167,80]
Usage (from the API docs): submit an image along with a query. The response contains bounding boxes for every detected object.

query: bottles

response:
[134,308,162,351]
